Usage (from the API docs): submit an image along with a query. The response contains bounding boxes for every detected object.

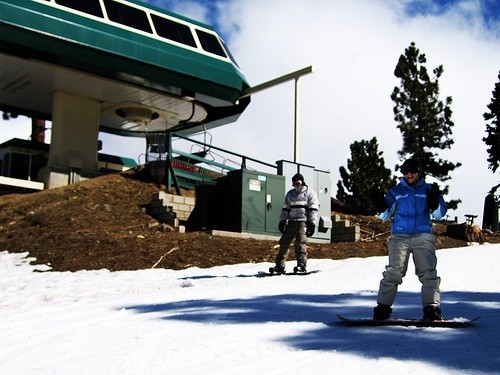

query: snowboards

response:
[335,313,483,329]
[253,269,321,279]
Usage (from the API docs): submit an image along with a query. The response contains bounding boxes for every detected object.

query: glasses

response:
[293,180,301,187]
[401,167,420,175]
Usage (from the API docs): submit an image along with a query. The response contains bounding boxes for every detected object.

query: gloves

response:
[371,192,388,214]
[425,182,440,210]
[306,225,315,237]
[278,219,287,234]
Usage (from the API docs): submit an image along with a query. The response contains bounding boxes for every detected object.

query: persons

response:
[269,174,318,273]
[374,159,447,318]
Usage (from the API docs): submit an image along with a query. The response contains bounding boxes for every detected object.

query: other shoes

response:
[373,305,392,319]
[423,306,442,320]
[269,266,285,272]
[294,266,306,273]
